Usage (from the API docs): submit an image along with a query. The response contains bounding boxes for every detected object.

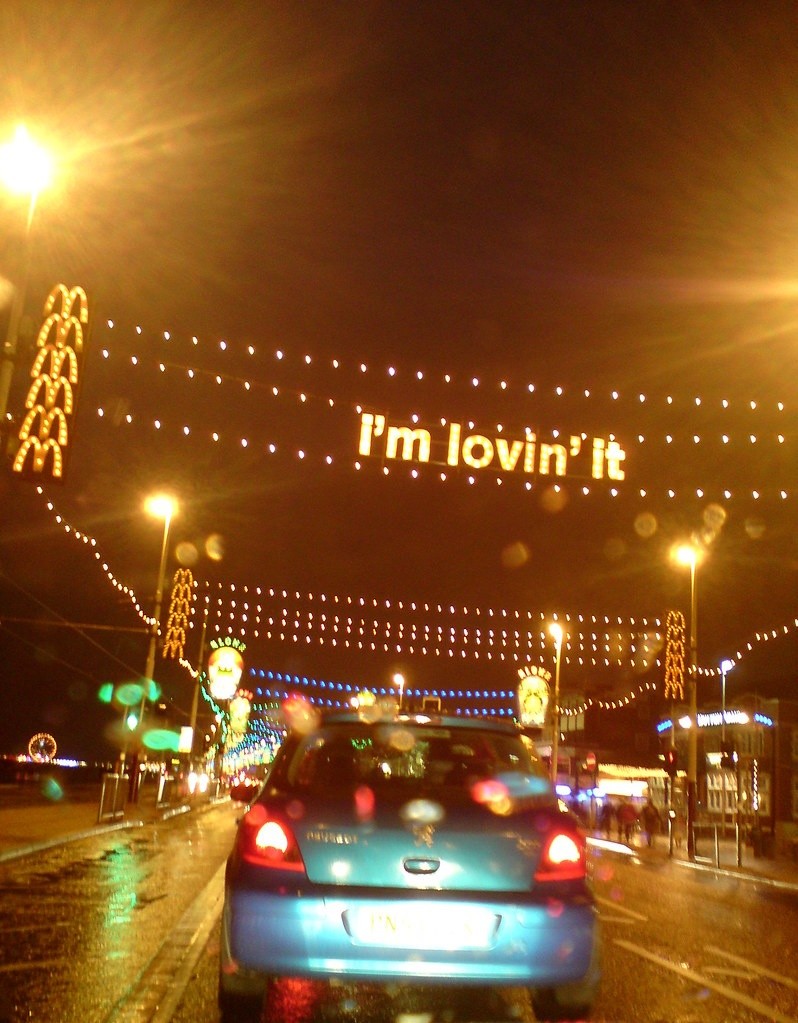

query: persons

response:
[615,803,637,842]
[599,800,614,836]
[642,799,663,846]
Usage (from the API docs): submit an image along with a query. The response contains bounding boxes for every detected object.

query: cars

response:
[218,710,595,995]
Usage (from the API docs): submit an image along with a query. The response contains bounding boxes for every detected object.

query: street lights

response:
[0,126,46,415]
[393,674,403,710]
[675,543,702,861]
[127,494,178,803]
[721,656,735,836]
[550,624,562,791]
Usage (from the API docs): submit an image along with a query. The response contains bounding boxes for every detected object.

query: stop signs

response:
[586,752,596,772]
